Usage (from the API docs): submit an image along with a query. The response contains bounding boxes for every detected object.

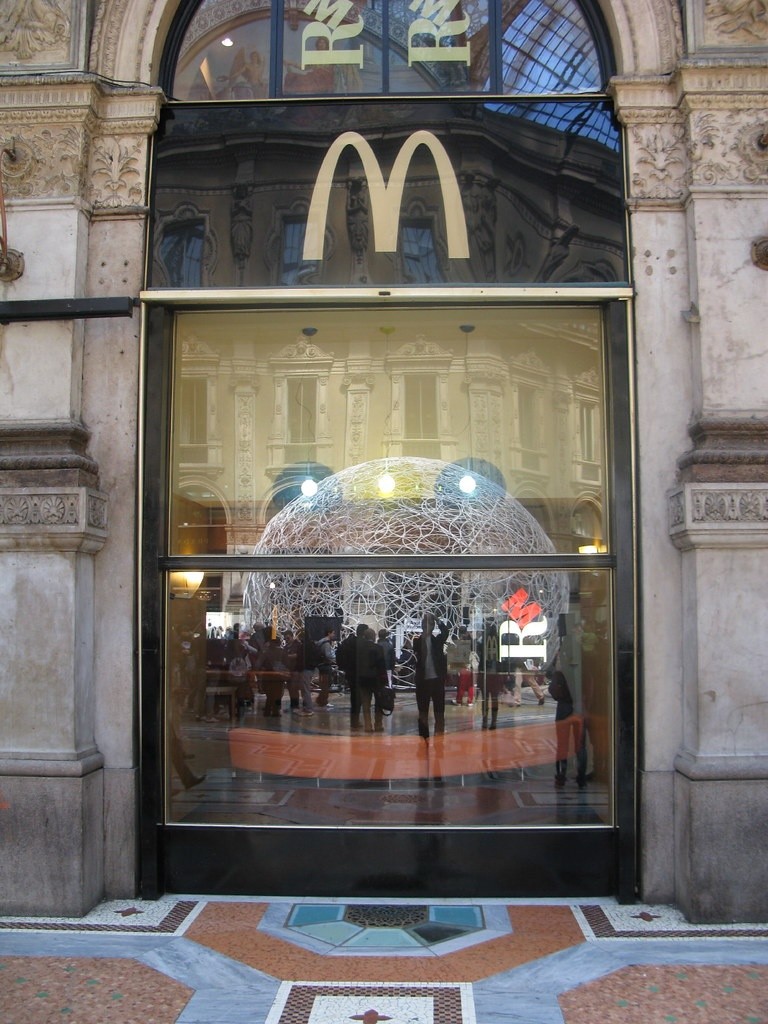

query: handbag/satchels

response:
[376,686,395,716]
[271,654,291,683]
[227,657,251,677]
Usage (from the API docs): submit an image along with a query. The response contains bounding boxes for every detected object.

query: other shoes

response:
[452,700,462,706]
[556,776,566,783]
[326,704,334,708]
[467,703,474,706]
[283,707,298,713]
[195,715,205,720]
[206,717,220,722]
[271,711,279,717]
[185,774,205,789]
[538,697,546,705]
[264,710,271,715]
[297,710,313,717]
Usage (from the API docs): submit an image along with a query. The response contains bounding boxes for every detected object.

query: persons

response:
[549,647,597,790]
[171,619,338,726]
[232,181,254,262]
[338,624,397,732]
[453,643,546,730]
[461,172,482,235]
[542,222,580,280]
[409,613,450,759]
[345,179,371,253]
[480,174,504,243]
[168,723,207,797]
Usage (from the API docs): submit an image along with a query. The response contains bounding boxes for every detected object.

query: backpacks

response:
[335,638,352,670]
[310,641,332,666]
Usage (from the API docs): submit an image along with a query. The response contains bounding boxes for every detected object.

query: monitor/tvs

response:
[305,617,341,641]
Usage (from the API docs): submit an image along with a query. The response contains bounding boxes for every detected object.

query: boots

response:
[482,708,488,728]
[491,708,499,730]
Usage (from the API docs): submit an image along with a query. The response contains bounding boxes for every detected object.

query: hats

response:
[253,623,265,631]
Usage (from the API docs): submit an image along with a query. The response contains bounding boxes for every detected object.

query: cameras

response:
[424,614,434,621]
